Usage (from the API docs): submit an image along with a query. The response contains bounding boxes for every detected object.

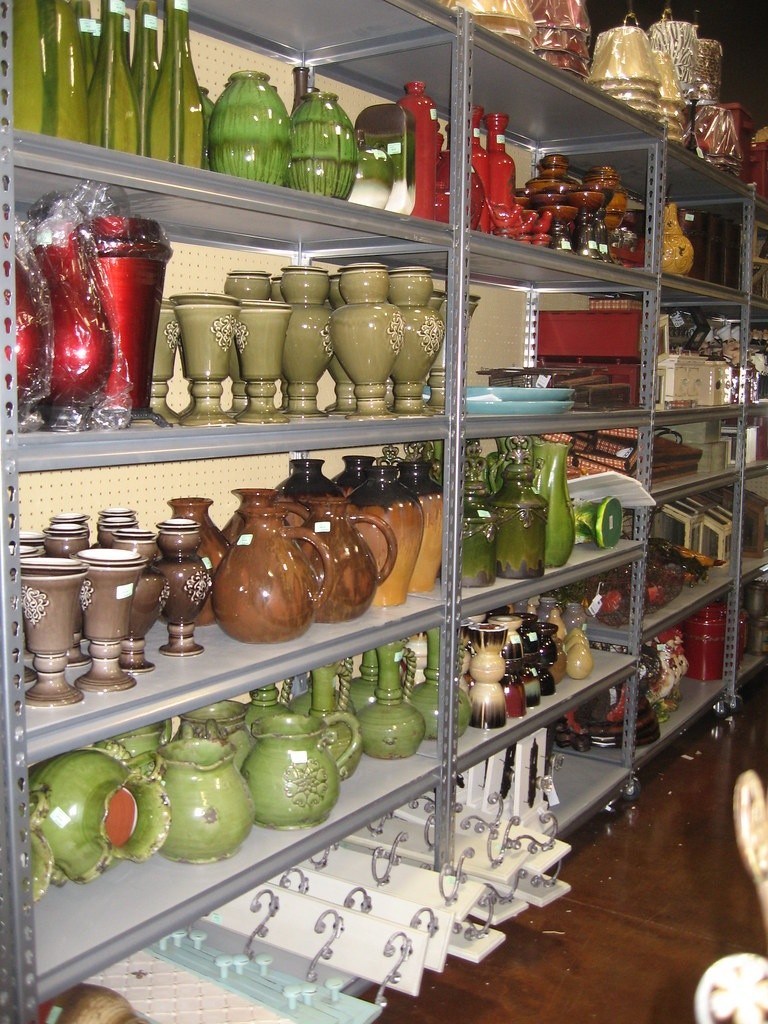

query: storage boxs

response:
[537,309,642,363]
[538,362,639,407]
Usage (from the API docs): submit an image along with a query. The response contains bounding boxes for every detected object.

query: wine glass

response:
[20,531,148,708]
[150,292,294,427]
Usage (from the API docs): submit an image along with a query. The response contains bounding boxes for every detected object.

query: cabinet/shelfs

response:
[0,0,768,1024]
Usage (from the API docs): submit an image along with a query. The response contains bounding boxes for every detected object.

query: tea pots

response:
[549,216,572,236]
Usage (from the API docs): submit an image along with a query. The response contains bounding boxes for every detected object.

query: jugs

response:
[93,699,364,862]
[168,486,398,645]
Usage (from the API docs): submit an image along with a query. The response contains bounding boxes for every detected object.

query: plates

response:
[426,379,575,413]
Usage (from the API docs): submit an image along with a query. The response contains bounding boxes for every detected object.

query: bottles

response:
[662,202,693,275]
[244,626,473,760]
[4,218,117,431]
[198,67,394,210]
[379,429,577,589]
[274,453,442,607]
[406,596,593,729]
[224,262,446,419]
[396,81,516,234]
[42,507,212,674]
[574,207,617,261]
[26,723,170,903]
[13,0,205,170]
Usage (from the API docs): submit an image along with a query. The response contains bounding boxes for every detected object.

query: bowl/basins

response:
[435,0,687,146]
[515,179,604,221]
